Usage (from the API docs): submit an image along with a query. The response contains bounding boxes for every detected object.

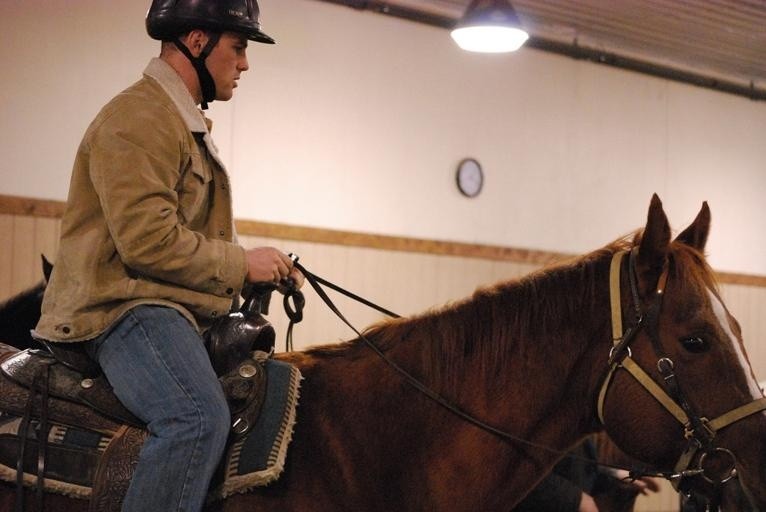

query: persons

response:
[29,0,303,512]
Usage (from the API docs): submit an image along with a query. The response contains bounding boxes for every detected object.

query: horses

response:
[0,185,766,511]
[0,253,55,350]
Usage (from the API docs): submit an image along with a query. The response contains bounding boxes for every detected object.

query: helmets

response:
[145,0,276,44]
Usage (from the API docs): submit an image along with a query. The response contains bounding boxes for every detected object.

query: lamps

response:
[448,0,529,54]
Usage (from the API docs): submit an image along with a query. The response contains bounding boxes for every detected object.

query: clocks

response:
[456,158,484,198]
